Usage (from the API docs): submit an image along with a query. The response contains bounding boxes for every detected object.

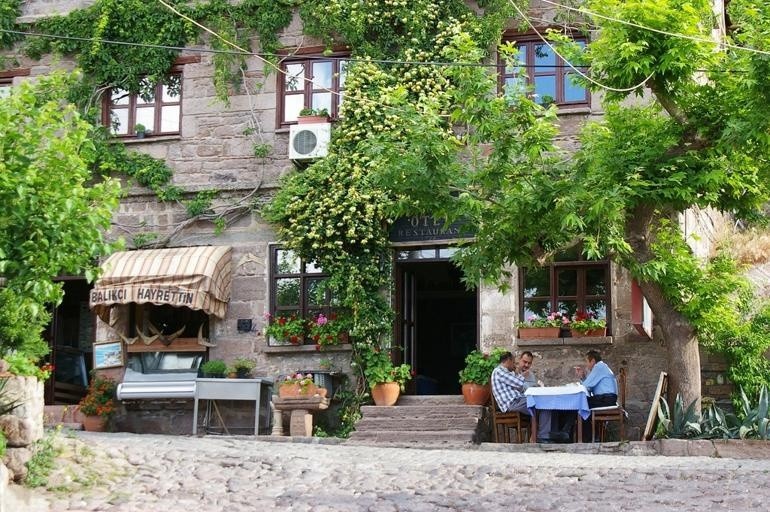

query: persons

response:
[491,352,559,443]
[558,350,618,443]
[510,351,536,393]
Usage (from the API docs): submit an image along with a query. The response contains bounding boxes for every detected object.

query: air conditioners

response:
[288,124,331,163]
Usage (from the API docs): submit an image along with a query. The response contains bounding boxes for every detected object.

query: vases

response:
[268,334,304,347]
[570,328,607,336]
[463,378,492,405]
[338,330,349,343]
[316,388,327,395]
[517,327,561,339]
[82,414,111,430]
[370,381,401,406]
[279,383,316,396]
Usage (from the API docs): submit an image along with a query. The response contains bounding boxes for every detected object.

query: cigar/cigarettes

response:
[574,367,580,368]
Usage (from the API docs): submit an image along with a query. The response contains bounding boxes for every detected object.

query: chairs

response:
[591,368,625,441]
[491,394,529,442]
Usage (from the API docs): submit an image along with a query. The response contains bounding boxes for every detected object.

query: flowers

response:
[79,369,118,416]
[568,302,607,334]
[459,346,507,386]
[264,313,306,344]
[307,312,351,353]
[279,374,313,395]
[514,311,571,328]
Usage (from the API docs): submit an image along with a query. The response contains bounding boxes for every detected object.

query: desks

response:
[524,385,591,443]
[193,377,274,435]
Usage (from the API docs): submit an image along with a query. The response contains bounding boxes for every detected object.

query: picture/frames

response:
[93,339,124,369]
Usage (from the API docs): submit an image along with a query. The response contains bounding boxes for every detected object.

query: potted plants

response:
[232,357,255,379]
[228,367,239,378]
[320,359,334,369]
[298,108,330,123]
[135,124,145,139]
[365,350,417,393]
[201,359,227,378]
